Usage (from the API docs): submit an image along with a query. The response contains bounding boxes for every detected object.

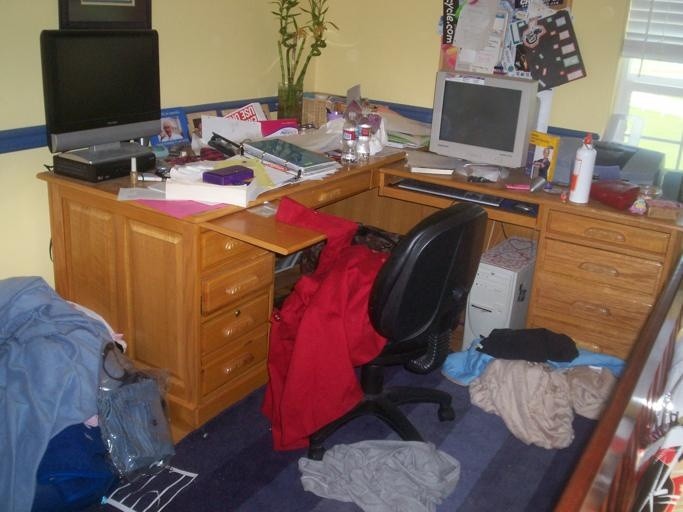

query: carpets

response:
[67,352,595,511]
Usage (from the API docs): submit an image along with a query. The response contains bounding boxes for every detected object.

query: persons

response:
[161,119,183,142]
[533,147,550,181]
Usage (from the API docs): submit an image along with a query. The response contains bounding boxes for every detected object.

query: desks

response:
[36,143,428,431]
[378,157,682,363]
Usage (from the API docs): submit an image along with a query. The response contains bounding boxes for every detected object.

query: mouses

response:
[513,201,534,213]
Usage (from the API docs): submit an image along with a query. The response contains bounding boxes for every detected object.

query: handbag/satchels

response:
[93,377,176,482]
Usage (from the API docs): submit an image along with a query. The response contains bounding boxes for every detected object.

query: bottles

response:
[569,132,597,203]
[355,110,373,161]
[341,110,357,163]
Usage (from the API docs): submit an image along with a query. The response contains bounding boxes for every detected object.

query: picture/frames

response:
[150,110,190,145]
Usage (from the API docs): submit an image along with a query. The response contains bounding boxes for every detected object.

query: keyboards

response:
[387,177,504,208]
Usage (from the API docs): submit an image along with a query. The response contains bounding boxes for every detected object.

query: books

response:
[165,175,258,208]
[404,150,454,176]
[240,151,342,178]
[644,198,680,222]
[242,138,335,175]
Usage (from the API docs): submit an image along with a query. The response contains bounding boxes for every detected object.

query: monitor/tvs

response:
[428,70,540,181]
[40,29,161,165]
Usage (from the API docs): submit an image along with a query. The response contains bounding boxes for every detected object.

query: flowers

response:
[269,0,339,125]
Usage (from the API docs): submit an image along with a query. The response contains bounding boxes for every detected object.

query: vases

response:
[276,83,303,120]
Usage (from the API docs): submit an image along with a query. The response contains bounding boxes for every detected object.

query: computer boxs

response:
[462,234,537,352]
[53,153,156,183]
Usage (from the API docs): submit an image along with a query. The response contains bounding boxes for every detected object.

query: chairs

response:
[276,204,488,462]
